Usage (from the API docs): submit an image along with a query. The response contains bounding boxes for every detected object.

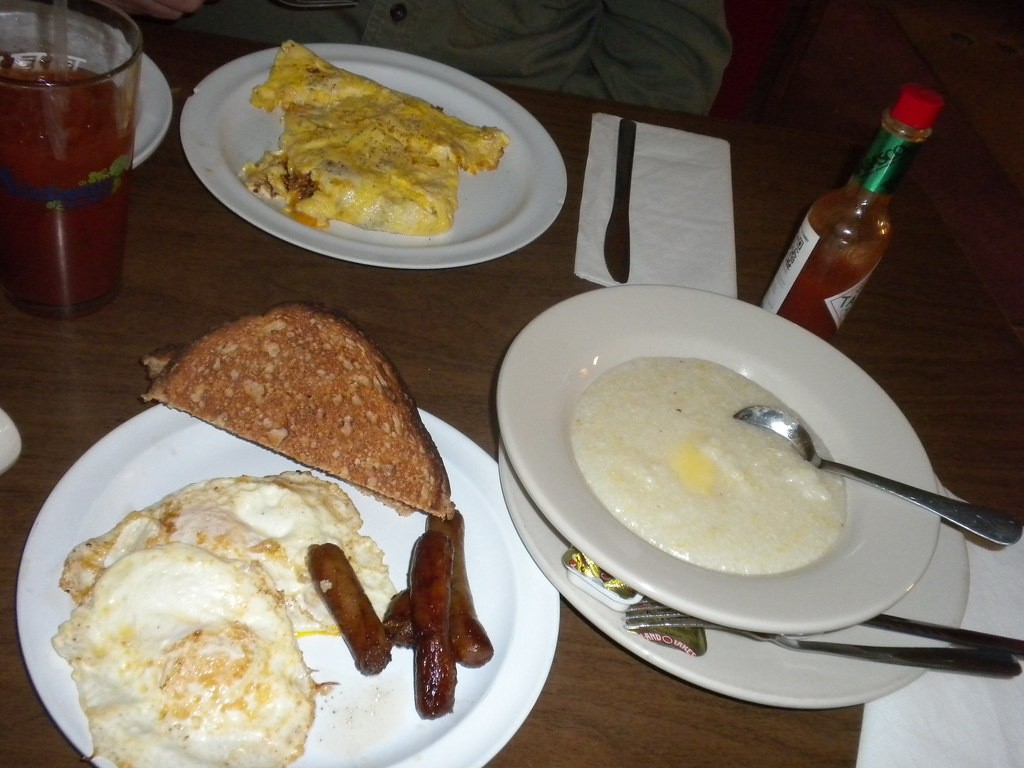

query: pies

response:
[233,39,508,238]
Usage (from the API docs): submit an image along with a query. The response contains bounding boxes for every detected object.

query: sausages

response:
[304,508,493,719]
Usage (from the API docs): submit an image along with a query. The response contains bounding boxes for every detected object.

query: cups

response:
[1,0,144,321]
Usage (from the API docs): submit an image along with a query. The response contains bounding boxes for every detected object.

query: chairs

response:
[702,0,829,121]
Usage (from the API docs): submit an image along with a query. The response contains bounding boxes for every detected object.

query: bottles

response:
[759,84,944,344]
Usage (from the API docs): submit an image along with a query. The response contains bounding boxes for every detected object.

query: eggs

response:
[50,470,395,768]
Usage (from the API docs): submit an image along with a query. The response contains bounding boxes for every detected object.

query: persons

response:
[103,0,735,115]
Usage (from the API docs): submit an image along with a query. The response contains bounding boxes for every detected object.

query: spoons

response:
[736,408,1024,544]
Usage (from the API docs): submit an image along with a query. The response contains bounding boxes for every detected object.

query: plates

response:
[1,11,173,171]
[497,433,970,710]
[180,44,567,269]
[18,402,560,768]
[496,283,942,633]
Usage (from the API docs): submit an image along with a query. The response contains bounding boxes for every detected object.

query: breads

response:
[134,300,459,522]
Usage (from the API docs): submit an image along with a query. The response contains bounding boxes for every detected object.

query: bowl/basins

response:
[562,549,645,612]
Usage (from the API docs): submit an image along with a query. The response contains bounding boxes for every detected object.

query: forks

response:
[617,598,1022,679]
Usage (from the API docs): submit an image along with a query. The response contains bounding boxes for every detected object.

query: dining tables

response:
[0,0,1023,766]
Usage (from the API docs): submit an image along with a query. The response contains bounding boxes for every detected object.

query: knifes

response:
[604,118,636,283]
